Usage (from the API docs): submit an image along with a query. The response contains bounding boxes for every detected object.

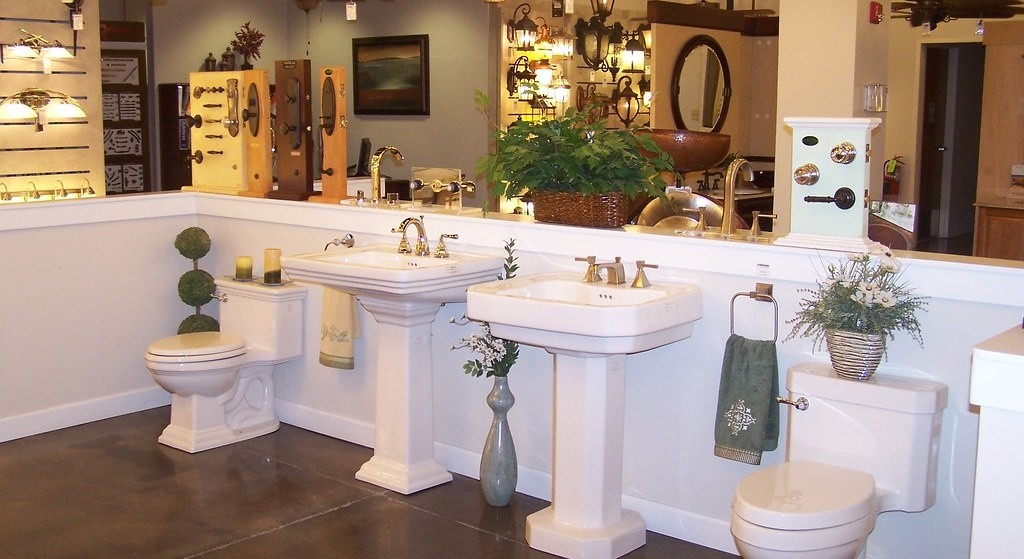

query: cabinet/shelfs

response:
[973,202,1024,260]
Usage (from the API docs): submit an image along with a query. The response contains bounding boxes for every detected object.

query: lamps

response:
[505,1,653,129]
[5,29,75,61]
[0,89,86,117]
[0,178,97,202]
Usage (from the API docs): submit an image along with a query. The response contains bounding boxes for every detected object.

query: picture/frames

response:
[352,33,430,116]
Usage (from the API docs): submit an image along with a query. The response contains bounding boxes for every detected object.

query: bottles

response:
[222,47,235,70]
[205,52,216,71]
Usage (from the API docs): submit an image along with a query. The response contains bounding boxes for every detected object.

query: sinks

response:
[278,241,506,303]
[465,269,704,353]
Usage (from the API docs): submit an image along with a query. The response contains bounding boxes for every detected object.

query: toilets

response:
[730,360,950,559]
[143,274,308,454]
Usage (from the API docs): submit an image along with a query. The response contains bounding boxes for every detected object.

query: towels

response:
[712,334,781,466]
[318,284,362,370]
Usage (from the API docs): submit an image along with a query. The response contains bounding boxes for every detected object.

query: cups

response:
[264,248,281,284]
[235,256,253,279]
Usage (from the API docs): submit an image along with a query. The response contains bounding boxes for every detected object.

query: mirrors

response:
[672,33,732,136]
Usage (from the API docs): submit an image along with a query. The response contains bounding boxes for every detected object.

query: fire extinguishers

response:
[882,155,906,202]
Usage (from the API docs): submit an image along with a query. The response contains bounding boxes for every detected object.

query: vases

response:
[480,375,517,507]
[824,327,887,380]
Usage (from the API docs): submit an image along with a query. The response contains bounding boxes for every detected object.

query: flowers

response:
[778,246,930,362]
[448,236,520,378]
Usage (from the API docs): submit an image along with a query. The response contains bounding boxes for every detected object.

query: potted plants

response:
[230,19,265,71]
[475,88,684,225]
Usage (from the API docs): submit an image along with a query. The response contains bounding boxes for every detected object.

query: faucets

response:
[390,217,431,257]
[591,255,625,287]
[720,158,753,240]
[368,144,405,205]
